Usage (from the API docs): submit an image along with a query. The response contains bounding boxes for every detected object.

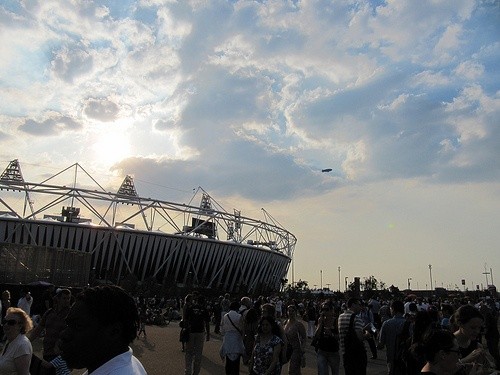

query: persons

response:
[180,291,500,375]
[40,340,72,375]
[0,308,33,375]
[58,285,147,375]
[30,289,71,375]
[138,304,146,337]
[17,289,33,315]
[0,288,11,344]
[138,293,183,324]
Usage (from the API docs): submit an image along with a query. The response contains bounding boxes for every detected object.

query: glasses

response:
[2,318,20,325]
[446,348,460,356]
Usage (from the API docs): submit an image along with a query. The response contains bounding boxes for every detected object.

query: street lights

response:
[345,277,348,290]
[428,264,433,290]
[338,266,340,292]
[408,278,412,289]
[320,270,322,289]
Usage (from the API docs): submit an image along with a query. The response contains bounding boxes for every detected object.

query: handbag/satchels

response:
[301,353,306,368]
[343,328,367,370]
[280,342,294,365]
[388,340,419,375]
[319,335,339,352]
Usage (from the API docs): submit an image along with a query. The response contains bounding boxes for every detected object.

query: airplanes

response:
[322,169,332,172]
